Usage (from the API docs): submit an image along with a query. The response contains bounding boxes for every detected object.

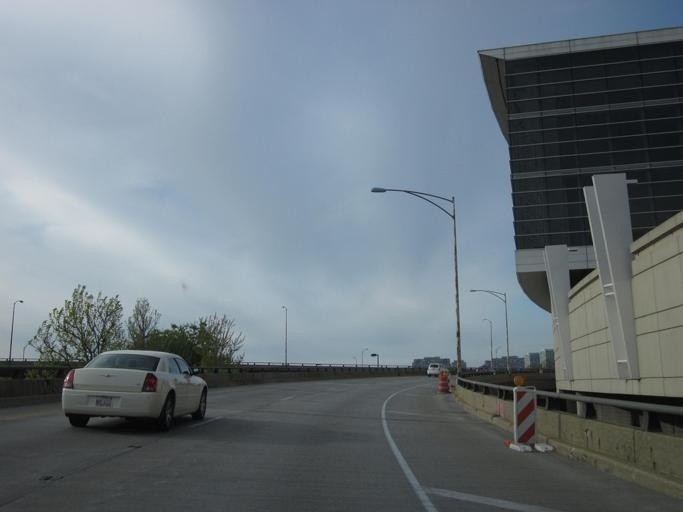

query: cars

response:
[61,350,208,432]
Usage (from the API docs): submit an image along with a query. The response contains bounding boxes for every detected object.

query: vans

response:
[426,362,448,377]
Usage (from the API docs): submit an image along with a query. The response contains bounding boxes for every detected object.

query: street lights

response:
[280,305,286,365]
[361,348,379,367]
[370,186,462,376]
[469,290,510,375]
[9,300,23,361]
